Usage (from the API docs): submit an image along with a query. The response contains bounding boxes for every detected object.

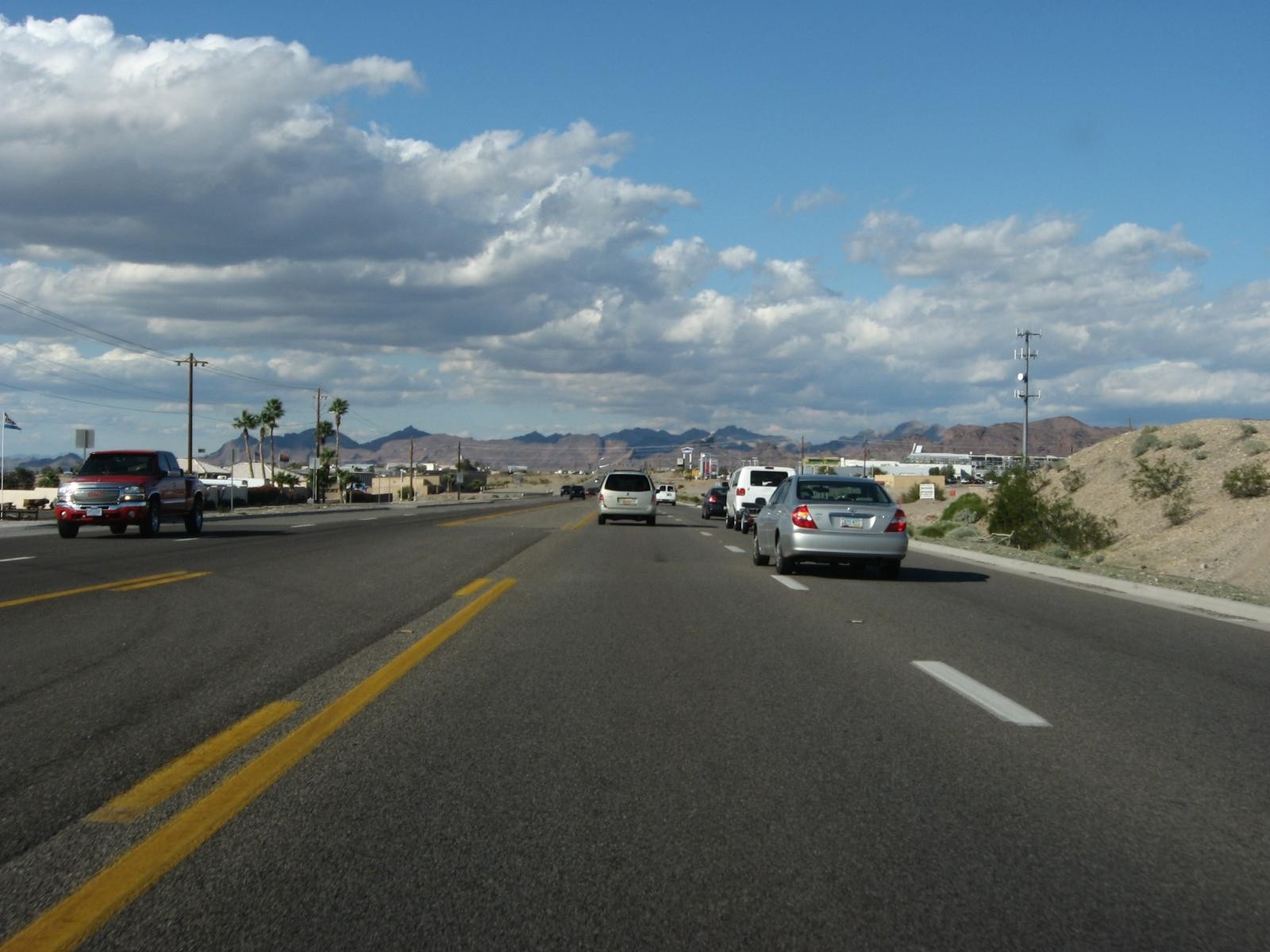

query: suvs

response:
[570,486,585,500]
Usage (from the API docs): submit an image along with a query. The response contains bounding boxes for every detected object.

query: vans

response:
[655,484,678,506]
[721,465,796,534]
[596,469,657,526]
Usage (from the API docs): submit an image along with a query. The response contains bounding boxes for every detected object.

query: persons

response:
[98,458,115,473]
[803,489,813,496]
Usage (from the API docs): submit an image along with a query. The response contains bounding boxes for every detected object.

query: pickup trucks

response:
[53,448,207,539]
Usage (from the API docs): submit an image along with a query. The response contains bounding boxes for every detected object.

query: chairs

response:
[841,493,867,502]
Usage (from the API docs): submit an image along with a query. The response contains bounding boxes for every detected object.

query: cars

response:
[701,488,729,520]
[752,475,909,581]
[946,472,998,485]
[560,486,571,496]
